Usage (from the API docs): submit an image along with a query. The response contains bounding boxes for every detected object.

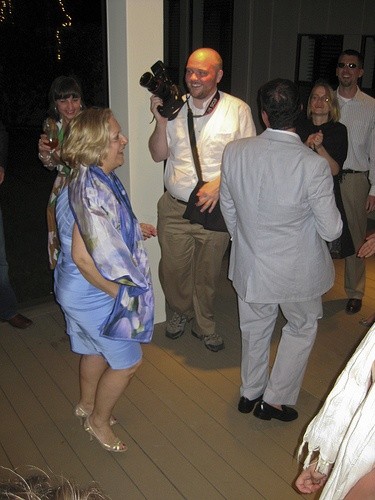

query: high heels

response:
[75,403,118,427]
[83,417,127,453]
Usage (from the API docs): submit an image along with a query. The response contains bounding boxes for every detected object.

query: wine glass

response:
[44,119,58,168]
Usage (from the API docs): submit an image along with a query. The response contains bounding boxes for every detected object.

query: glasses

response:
[337,62,359,69]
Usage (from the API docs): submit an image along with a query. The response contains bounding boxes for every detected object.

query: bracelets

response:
[39,153,51,161]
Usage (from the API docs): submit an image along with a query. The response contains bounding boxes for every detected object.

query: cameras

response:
[142,61,183,116]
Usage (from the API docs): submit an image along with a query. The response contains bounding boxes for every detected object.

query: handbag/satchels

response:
[181,180,229,232]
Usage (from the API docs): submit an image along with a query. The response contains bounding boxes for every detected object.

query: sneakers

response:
[192,327,225,352]
[166,310,193,339]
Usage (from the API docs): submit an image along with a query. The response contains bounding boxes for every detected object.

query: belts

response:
[341,168,361,173]
[170,196,188,205]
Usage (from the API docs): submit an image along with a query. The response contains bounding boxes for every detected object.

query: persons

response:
[0,121,33,330]
[296,321,375,500]
[38,78,90,271]
[328,49,375,314]
[149,47,256,352]
[53,107,154,452]
[298,84,356,260]
[220,80,343,423]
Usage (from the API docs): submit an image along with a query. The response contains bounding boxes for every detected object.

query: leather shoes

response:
[0,313,32,329]
[252,400,298,422]
[346,298,363,314]
[238,394,264,412]
